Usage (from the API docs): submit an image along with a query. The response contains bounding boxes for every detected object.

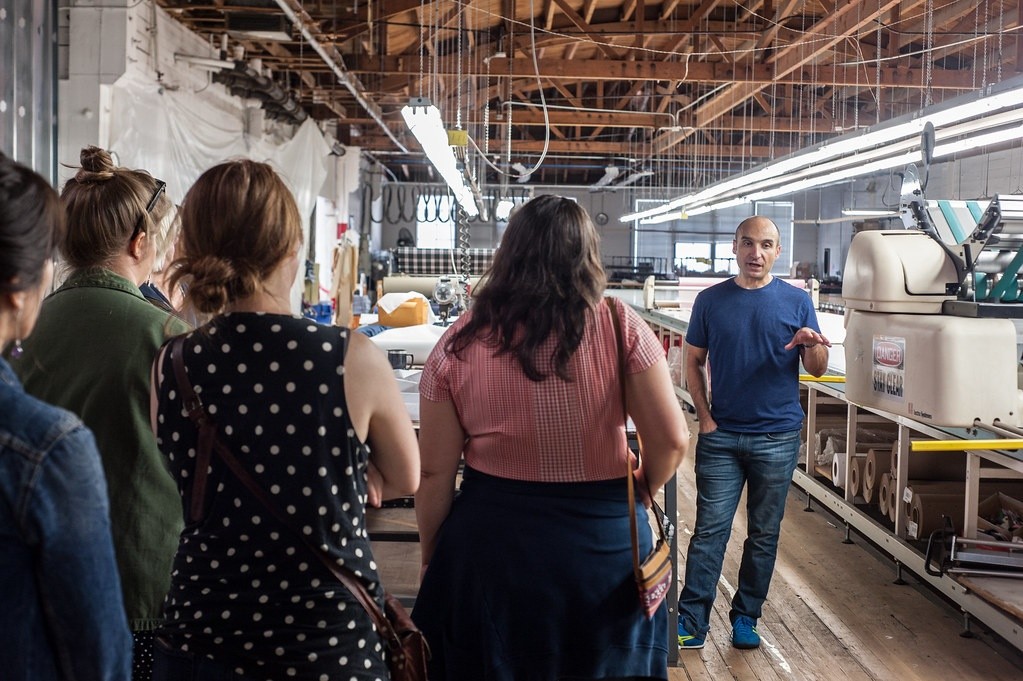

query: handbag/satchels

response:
[636,539,673,621]
[377,587,429,681]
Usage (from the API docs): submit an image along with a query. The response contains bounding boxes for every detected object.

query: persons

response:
[0,150,133,681]
[2,146,209,681]
[410,194,689,681]
[678,217,833,649]
[150,159,422,681]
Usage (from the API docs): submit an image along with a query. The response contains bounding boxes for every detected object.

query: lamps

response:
[615,0,1023,226]
[400,0,488,216]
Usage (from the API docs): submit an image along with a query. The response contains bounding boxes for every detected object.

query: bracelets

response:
[804,343,817,348]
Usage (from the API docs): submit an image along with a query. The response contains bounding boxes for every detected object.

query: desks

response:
[603,289,1023,652]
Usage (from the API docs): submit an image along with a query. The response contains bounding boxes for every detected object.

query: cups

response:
[386,348,414,370]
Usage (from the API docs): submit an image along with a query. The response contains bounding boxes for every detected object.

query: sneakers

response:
[677,620,707,649]
[728,609,761,649]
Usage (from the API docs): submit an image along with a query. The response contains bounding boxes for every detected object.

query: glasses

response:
[131,177,167,243]
